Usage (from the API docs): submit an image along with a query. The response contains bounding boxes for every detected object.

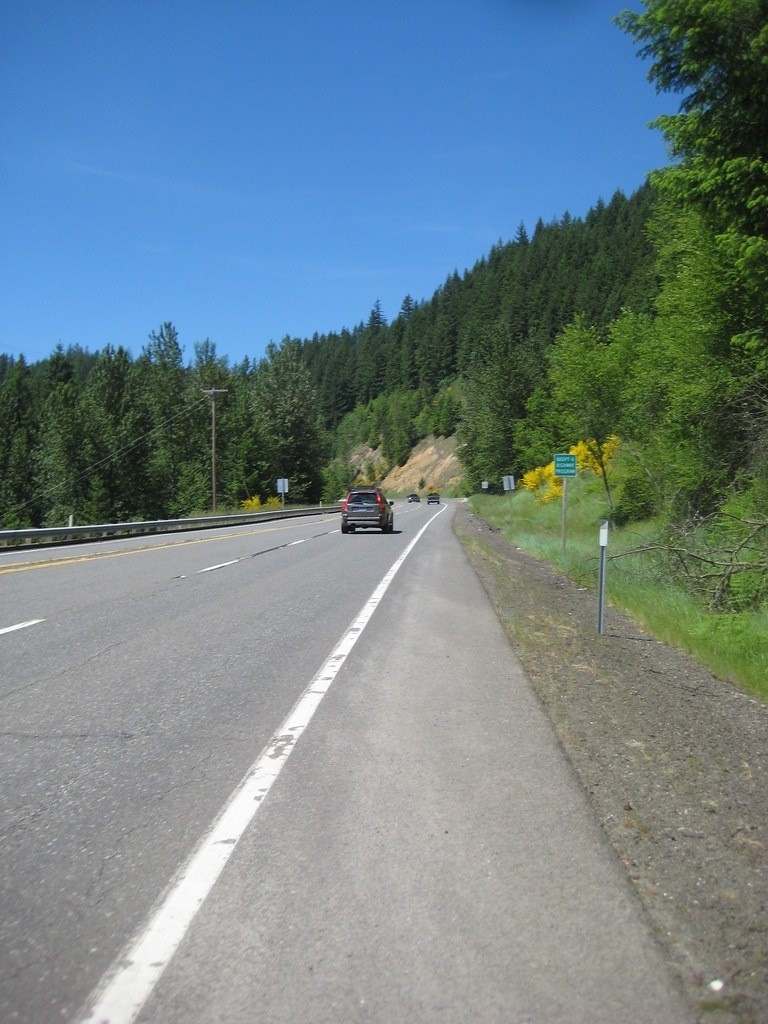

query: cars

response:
[406,494,420,503]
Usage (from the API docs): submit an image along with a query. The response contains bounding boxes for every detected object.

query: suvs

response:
[426,493,441,504]
[340,487,395,534]
